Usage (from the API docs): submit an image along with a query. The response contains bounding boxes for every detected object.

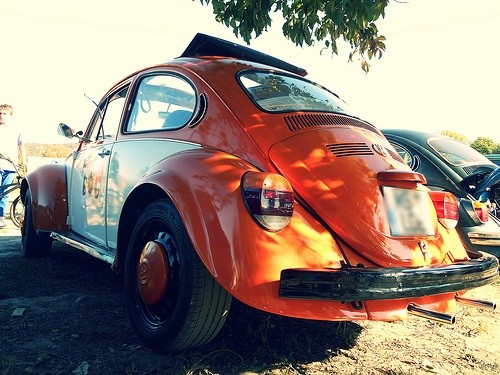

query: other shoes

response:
[0,217,6,226]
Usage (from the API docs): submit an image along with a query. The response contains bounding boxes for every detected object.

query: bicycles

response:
[0,156,28,228]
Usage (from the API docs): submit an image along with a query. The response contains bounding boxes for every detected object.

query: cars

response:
[20,33,499,355]
[378,127,499,217]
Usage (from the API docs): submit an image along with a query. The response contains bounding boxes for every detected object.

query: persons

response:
[0,104,30,227]
